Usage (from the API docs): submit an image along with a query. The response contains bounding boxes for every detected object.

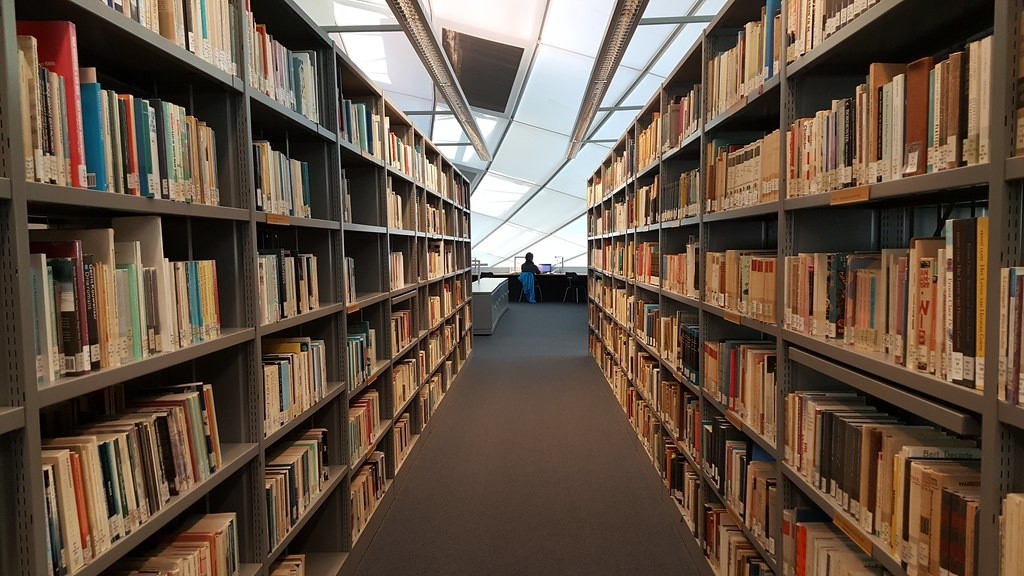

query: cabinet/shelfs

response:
[0,0,471,576]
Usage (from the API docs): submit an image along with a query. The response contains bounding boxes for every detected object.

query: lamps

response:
[385,0,494,163]
[587,0,1024,576]
[563,0,650,161]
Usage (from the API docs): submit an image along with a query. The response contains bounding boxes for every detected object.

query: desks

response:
[471,277,509,336]
[511,271,587,304]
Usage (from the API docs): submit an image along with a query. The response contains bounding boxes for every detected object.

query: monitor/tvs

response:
[539,264,551,273]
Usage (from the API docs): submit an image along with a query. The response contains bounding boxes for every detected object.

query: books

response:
[586,0,1023,576]
[19,1,471,576]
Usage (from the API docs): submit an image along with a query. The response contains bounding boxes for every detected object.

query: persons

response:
[522,253,541,291]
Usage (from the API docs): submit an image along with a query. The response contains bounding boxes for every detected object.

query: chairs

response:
[517,272,543,303]
[562,271,587,303]
